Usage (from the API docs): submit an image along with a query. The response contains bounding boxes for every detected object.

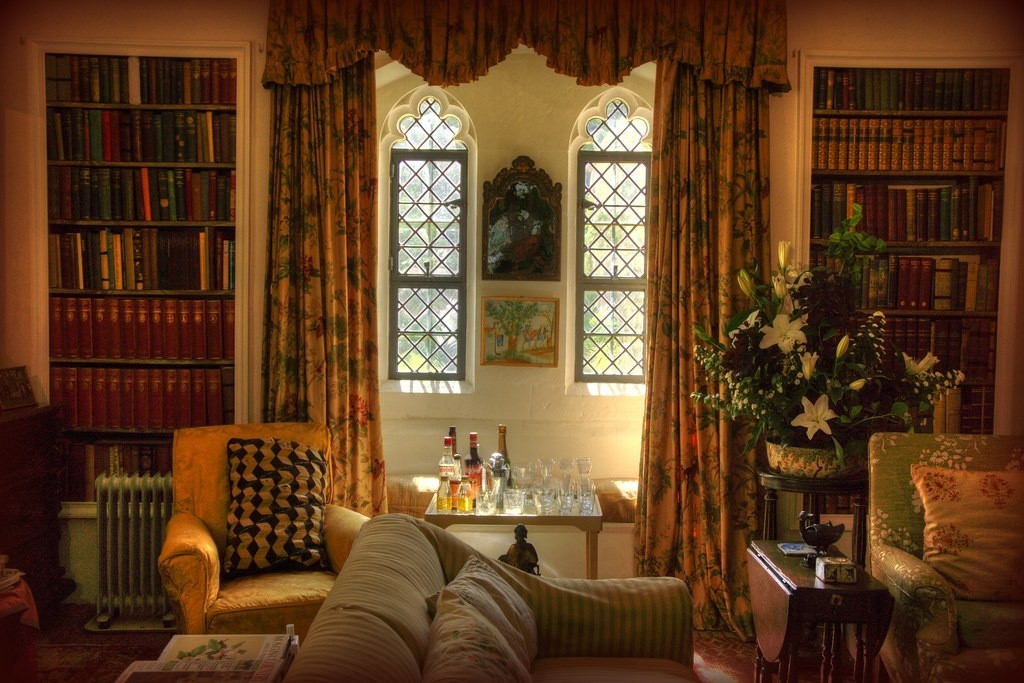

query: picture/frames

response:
[0,365,39,411]
[481,296,560,367]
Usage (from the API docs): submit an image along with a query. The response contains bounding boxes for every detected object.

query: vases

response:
[765,438,869,478]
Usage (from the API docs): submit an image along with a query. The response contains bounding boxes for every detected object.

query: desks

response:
[746,540,897,683]
[116,624,300,683]
[753,472,870,683]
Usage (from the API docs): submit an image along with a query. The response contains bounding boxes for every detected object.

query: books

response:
[811,67,1010,171]
[113,634,294,683]
[810,177,1004,435]
[44,52,236,501]
[817,495,869,515]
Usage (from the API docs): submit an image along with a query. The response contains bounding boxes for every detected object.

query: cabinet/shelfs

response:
[0,404,60,581]
[44,50,240,505]
[802,64,1010,532]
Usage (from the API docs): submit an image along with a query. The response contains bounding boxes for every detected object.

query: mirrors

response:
[482,156,562,282]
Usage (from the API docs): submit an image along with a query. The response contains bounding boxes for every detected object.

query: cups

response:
[502,490,524,514]
[476,485,495,514]
[511,457,595,515]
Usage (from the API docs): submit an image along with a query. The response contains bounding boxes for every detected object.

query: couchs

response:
[863,433,1024,683]
[385,473,639,523]
[158,423,371,648]
[284,513,703,683]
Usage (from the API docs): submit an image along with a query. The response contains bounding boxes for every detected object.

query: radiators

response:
[94,470,175,629]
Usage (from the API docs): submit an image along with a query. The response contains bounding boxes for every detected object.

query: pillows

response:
[221,437,327,582]
[910,464,1024,601]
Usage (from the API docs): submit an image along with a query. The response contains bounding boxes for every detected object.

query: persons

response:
[498,524,539,575]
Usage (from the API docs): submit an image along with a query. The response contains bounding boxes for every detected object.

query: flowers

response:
[689,202,967,472]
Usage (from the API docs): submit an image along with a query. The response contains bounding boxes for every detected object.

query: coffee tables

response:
[424,489,604,580]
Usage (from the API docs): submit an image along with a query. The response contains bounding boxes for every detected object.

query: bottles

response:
[436,424,511,512]
